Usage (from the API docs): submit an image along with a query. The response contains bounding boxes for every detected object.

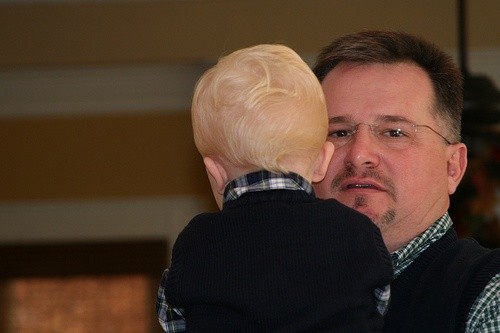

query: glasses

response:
[326,117,452,147]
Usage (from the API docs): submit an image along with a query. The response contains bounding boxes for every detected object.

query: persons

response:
[310,30,500,333]
[151,45,392,333]
